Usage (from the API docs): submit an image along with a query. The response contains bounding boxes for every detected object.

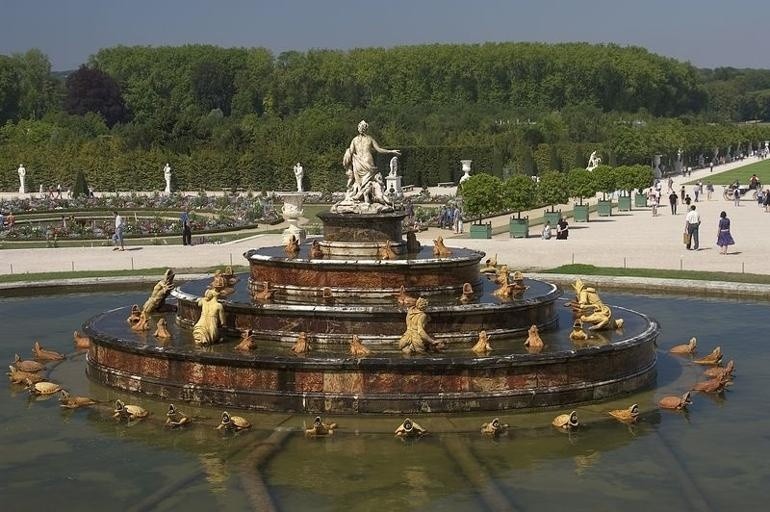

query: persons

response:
[293,162,305,193]
[641,142,770,216]
[190,286,223,344]
[437,204,464,233]
[542,221,553,241]
[556,219,569,240]
[398,202,406,228]
[397,295,441,356]
[344,166,354,191]
[715,211,735,255]
[112,211,126,252]
[560,219,568,239]
[684,205,701,251]
[406,201,415,226]
[180,207,193,245]
[571,278,624,332]
[330,120,401,215]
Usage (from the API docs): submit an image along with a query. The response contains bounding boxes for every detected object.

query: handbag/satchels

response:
[684,233,691,245]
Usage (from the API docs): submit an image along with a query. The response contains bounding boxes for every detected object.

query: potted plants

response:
[456,163,654,240]
